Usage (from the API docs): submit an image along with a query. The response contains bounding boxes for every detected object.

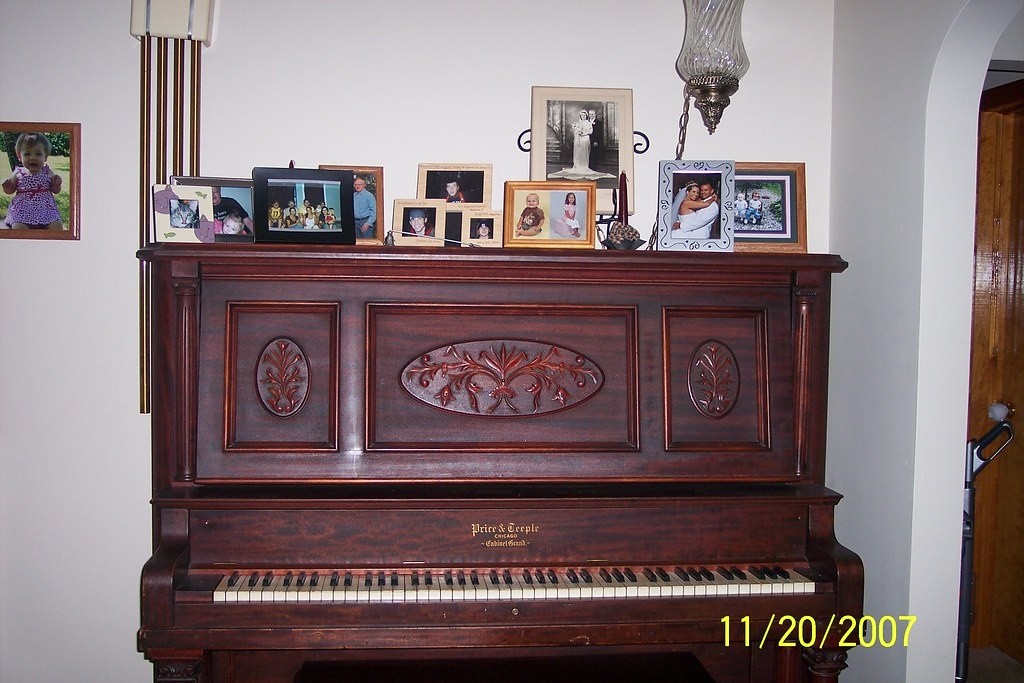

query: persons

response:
[212,186,254,236]
[268,198,338,230]
[406,110,765,239]
[1,133,64,231]
[352,176,377,239]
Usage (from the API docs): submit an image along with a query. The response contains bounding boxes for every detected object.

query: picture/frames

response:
[391,198,446,248]
[418,162,494,212]
[529,86,636,216]
[459,210,503,248]
[502,180,598,250]
[0,121,81,241]
[319,165,385,246]
[152,184,215,244]
[169,175,254,244]
[658,160,735,253]
[251,167,357,247]
[733,161,808,254]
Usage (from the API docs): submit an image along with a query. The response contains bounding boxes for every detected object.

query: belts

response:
[354,216,369,221]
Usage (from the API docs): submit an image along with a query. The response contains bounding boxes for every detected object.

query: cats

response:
[170,199,198,228]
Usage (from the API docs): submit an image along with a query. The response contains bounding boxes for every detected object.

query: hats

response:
[409,209,425,218]
[477,220,491,229]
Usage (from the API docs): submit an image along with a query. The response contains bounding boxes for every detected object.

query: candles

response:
[289,160,296,169]
[618,169,629,226]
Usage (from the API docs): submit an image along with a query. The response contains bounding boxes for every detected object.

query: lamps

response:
[646,0,751,252]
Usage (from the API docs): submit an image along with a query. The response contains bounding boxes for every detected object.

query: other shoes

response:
[753,218,756,223]
[744,219,748,224]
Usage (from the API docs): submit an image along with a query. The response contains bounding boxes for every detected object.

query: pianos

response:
[136,236,866,683]
